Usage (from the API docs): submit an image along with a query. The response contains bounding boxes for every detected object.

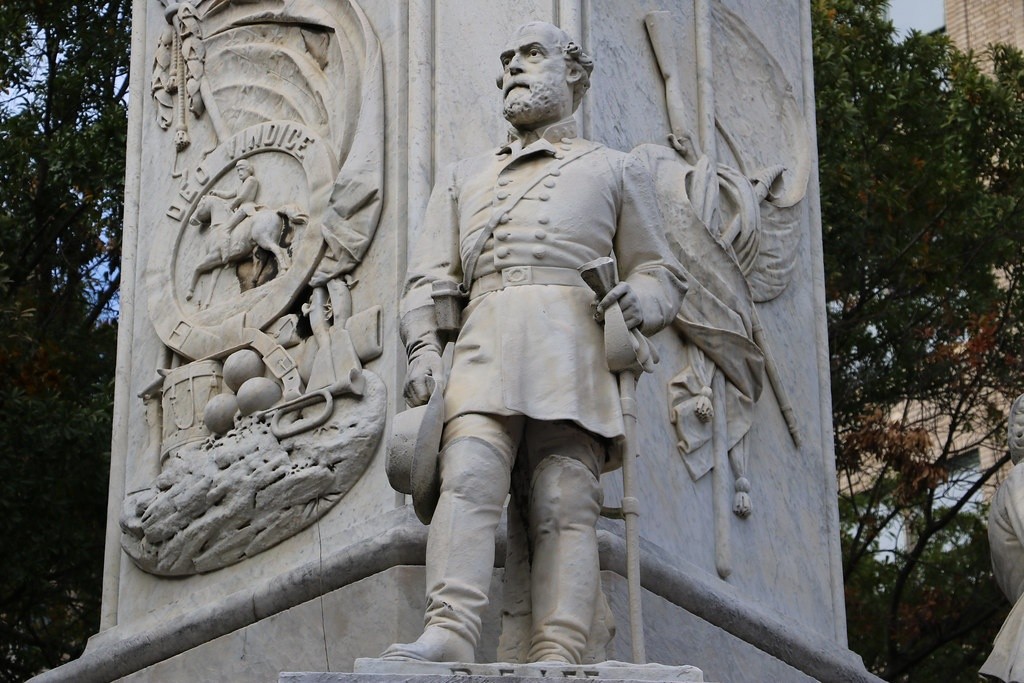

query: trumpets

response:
[256,367,369,438]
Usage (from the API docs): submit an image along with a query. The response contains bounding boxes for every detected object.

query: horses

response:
[186,194,309,301]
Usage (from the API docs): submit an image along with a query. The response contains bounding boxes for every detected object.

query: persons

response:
[207,159,258,265]
[380,20,690,667]
[978,393,1024,683]
